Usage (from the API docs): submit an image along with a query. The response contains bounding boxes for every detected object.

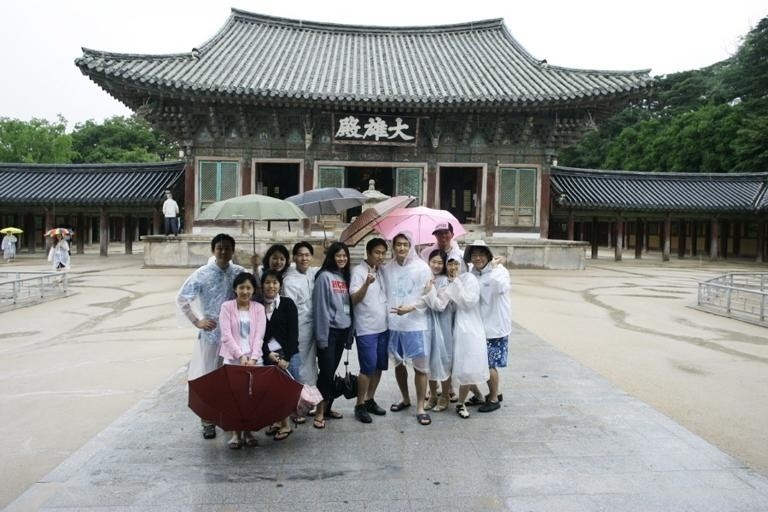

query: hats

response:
[462,240,492,262]
[432,221,453,234]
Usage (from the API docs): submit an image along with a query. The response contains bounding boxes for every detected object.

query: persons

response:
[281,242,320,424]
[349,237,390,424]
[47,234,71,284]
[1,231,17,263]
[421,249,453,412]
[314,241,355,428]
[260,244,292,296]
[383,231,434,425]
[219,272,266,450]
[254,269,300,441]
[161,193,180,236]
[177,233,263,439]
[445,254,490,418]
[463,239,513,413]
[419,221,471,403]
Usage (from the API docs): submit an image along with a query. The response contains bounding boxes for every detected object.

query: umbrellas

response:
[43,228,75,237]
[0,226,23,236]
[198,193,309,257]
[283,187,369,240]
[338,194,416,250]
[371,206,468,255]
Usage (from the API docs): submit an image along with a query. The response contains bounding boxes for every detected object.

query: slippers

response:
[267,410,342,440]
[390,401,410,412]
[228,436,241,448]
[246,438,258,446]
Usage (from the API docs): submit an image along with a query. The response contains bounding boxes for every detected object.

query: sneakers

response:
[203,424,216,438]
[417,390,502,424]
[365,399,386,415]
[353,404,371,424]
